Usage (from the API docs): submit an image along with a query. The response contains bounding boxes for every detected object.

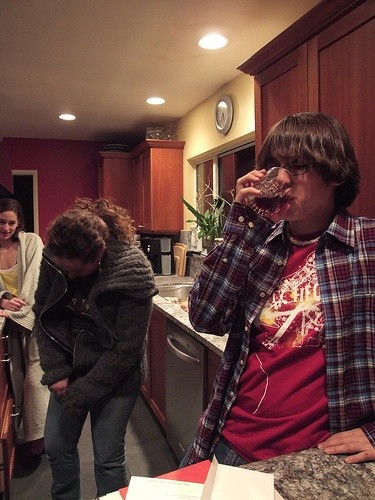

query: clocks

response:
[214,95,233,135]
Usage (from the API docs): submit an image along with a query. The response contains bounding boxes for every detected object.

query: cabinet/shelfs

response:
[237,0,375,221]
[139,307,236,462]
[96,139,186,233]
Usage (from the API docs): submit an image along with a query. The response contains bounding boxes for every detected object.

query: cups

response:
[180,230,192,251]
[247,167,296,217]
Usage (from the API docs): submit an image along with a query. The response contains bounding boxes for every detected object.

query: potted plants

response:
[181,192,228,252]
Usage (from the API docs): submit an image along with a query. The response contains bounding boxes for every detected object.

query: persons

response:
[0,198,47,457]
[32,197,159,500]
[176,113,375,464]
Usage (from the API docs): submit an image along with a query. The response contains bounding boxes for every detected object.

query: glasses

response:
[259,161,314,176]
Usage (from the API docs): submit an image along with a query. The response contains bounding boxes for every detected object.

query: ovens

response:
[165,318,207,462]
